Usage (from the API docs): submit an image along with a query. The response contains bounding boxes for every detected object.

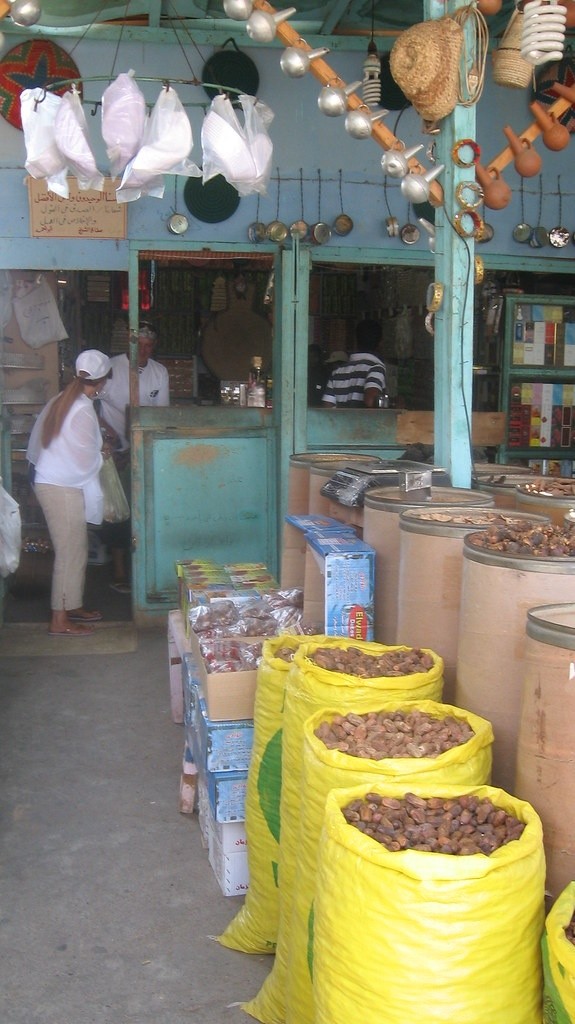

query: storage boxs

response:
[176,512,376,899]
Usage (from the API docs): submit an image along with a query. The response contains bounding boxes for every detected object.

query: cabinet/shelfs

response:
[471,294,574,476]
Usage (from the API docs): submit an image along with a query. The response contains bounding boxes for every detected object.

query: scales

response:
[319,462,453,505]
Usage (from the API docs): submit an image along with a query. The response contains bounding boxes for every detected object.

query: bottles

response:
[246,377,272,409]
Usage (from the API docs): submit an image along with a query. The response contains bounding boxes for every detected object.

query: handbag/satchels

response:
[12,275,69,349]
[102,452,130,526]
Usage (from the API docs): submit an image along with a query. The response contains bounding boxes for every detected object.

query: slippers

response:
[109,581,133,593]
[47,623,95,635]
[68,608,103,621]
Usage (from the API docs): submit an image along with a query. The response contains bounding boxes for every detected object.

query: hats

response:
[390,17,462,121]
[76,349,112,380]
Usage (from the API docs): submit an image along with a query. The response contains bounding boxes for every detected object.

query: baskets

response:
[491,9,535,89]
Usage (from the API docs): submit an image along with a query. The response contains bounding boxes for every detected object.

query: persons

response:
[25,349,114,636]
[321,333,387,409]
[94,322,170,592]
[308,343,331,408]
